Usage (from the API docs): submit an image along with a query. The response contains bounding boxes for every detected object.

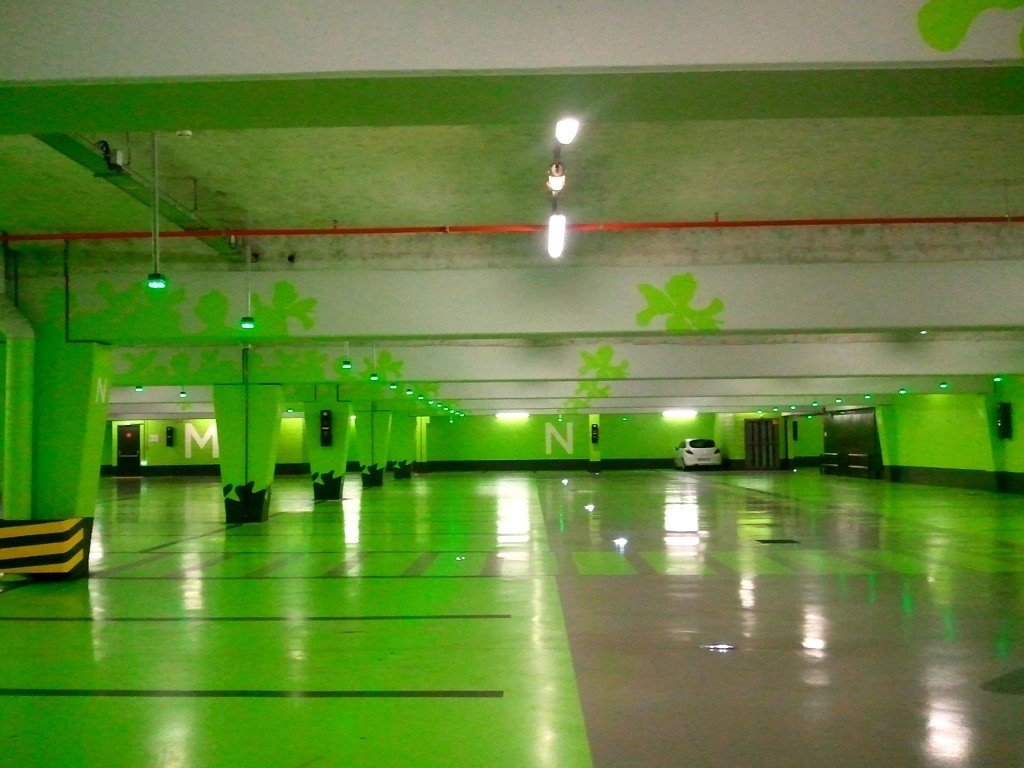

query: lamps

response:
[539,116,588,261]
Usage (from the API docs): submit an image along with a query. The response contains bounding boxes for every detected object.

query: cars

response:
[673,437,722,470]
